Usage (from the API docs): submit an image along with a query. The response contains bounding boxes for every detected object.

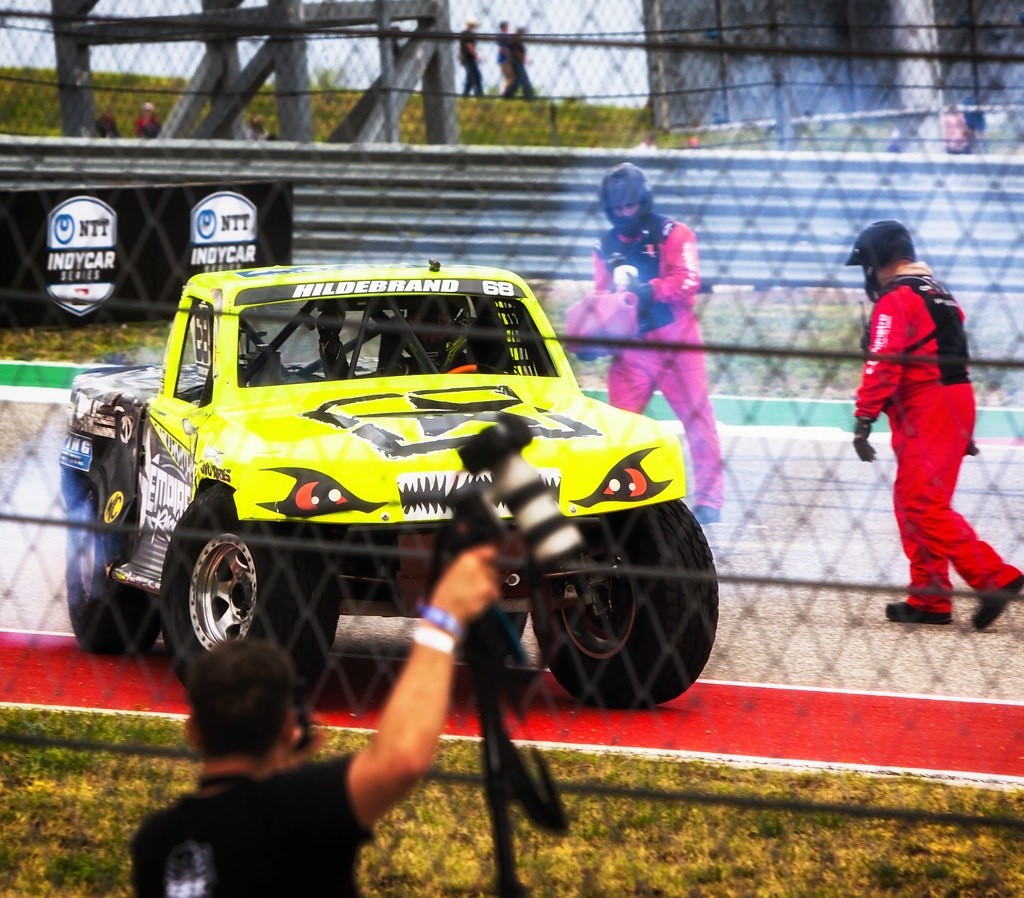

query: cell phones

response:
[289,681,312,752]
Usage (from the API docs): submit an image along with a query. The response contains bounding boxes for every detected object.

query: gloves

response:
[853,419,878,463]
[963,440,979,455]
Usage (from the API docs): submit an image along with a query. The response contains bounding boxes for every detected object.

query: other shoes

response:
[886,602,954,625]
[691,504,720,525]
[972,572,1024,628]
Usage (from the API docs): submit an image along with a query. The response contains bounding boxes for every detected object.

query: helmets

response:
[600,163,651,232]
[403,300,466,361]
[844,220,916,269]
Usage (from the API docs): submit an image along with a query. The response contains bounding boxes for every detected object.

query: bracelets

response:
[420,603,465,637]
[413,626,453,654]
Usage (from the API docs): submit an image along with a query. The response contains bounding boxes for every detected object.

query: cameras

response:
[458,416,583,563]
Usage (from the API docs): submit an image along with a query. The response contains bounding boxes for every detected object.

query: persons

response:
[945,106,986,154]
[496,21,535,97]
[843,220,1024,630]
[250,113,266,134]
[96,108,121,138]
[635,132,657,150]
[591,162,722,525]
[458,18,483,96]
[136,102,161,137]
[131,545,501,898]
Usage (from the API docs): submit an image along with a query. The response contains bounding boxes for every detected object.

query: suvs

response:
[66,256,721,713]
